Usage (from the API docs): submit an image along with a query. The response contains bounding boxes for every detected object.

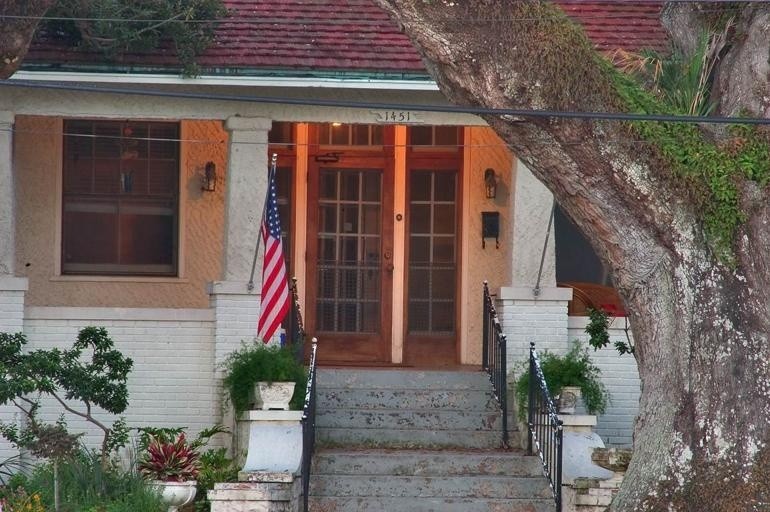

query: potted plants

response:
[511,339,613,423]
[213,336,309,417]
[136,431,201,512]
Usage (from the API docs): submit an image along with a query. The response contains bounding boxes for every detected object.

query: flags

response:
[257,166,294,345]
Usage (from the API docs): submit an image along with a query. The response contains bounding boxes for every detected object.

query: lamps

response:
[202,162,217,191]
[484,168,497,198]
